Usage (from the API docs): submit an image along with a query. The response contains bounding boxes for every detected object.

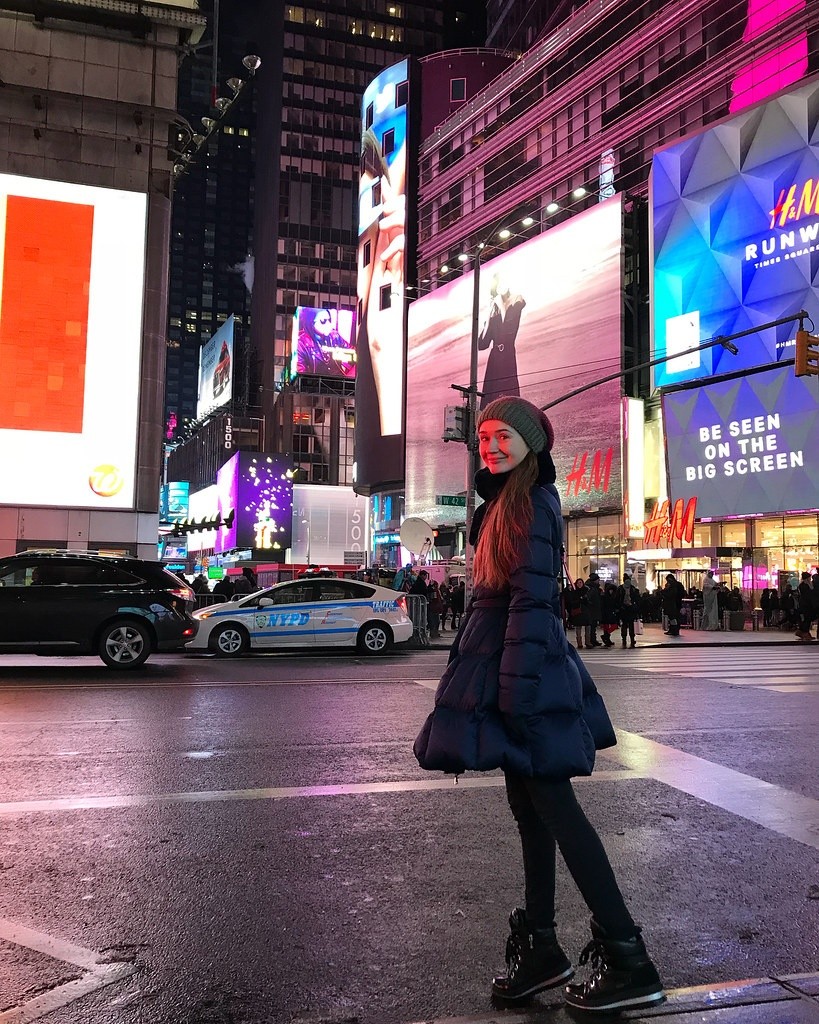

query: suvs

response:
[0,549,200,667]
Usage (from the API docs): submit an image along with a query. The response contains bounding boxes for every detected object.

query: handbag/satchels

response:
[571,608,582,617]
[633,619,644,635]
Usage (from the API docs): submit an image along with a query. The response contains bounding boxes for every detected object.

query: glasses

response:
[319,318,331,325]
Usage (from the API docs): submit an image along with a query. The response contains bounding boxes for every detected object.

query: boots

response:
[491,908,577,1002]
[562,915,668,1014]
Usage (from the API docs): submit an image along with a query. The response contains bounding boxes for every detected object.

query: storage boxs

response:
[723,609,746,631]
[692,611,702,630]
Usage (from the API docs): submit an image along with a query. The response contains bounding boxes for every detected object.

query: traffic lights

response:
[795,331,819,377]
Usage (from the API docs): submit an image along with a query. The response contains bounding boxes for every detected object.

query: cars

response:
[183,578,416,658]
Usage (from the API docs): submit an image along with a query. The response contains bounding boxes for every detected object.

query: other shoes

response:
[590,635,602,646]
[664,630,679,635]
[427,625,457,631]
[601,634,614,646]
[631,639,636,644]
[576,636,583,648]
[795,630,816,640]
[623,639,627,645]
[585,635,593,647]
[430,633,442,639]
[763,622,772,627]
[617,624,621,629]
[568,625,575,630]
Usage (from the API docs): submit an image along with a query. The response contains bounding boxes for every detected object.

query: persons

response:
[296,308,356,377]
[477,276,527,409]
[412,394,669,1013]
[188,565,817,649]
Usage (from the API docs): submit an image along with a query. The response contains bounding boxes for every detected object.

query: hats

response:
[802,572,811,580]
[477,396,555,455]
[623,573,631,582]
[588,573,599,581]
